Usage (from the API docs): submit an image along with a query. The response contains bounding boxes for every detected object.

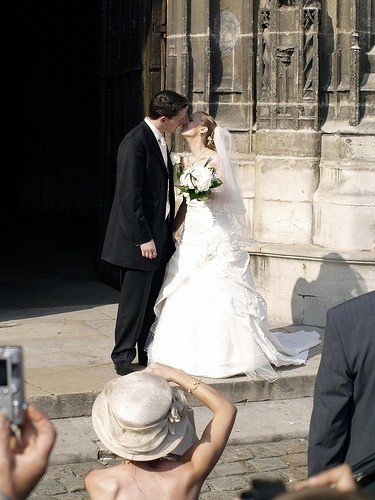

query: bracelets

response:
[189,379,204,394]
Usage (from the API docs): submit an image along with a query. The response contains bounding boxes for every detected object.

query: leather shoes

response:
[114,362,134,376]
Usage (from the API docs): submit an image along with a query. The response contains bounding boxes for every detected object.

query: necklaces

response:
[188,145,209,165]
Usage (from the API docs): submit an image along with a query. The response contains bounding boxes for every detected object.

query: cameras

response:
[0,344,28,424]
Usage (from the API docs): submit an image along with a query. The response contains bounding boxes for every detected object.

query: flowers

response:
[174,166,223,202]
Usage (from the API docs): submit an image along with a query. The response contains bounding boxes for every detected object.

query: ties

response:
[160,136,167,167]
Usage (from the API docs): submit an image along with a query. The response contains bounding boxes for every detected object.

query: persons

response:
[100,90,191,376]
[146,111,321,384]
[243,291,375,500]
[0,401,57,500]
[85,363,238,500]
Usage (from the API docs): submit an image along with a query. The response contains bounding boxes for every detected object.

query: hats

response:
[91,371,189,462]
